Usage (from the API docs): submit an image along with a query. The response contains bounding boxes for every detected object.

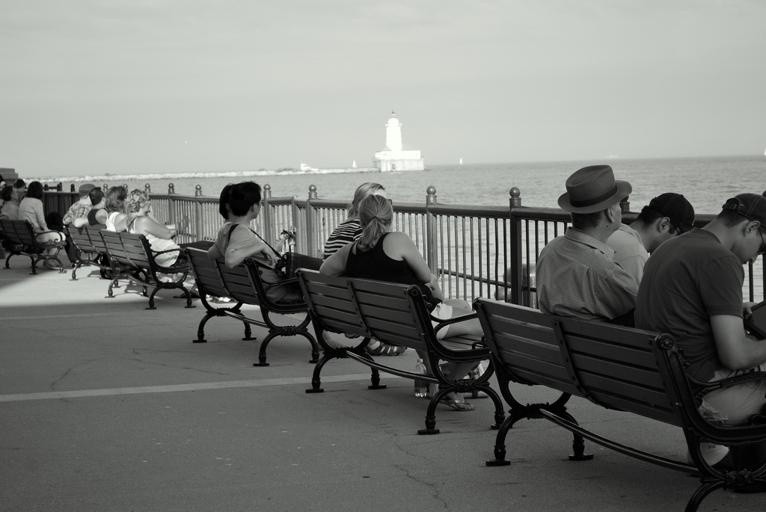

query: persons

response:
[631,191,763,493]
[0,177,232,304]
[318,194,486,413]
[323,180,474,400]
[217,183,235,225]
[207,181,408,358]
[537,165,639,334]
[597,193,695,295]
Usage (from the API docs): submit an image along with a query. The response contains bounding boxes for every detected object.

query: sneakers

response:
[71,261,88,267]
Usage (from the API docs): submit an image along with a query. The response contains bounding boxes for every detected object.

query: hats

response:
[722,193,766,227]
[650,193,694,229]
[558,165,632,214]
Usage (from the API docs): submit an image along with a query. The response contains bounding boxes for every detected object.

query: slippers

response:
[439,398,473,411]
[173,289,200,298]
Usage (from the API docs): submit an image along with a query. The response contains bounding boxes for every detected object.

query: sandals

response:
[367,341,406,356]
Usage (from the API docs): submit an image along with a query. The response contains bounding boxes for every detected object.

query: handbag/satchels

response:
[274,251,323,280]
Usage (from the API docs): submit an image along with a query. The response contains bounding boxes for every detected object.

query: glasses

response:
[757,230,766,254]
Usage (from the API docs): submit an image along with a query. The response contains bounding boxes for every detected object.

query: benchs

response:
[180,246,321,367]
[470,296,766,512]
[295,266,507,437]
[0,215,196,313]
